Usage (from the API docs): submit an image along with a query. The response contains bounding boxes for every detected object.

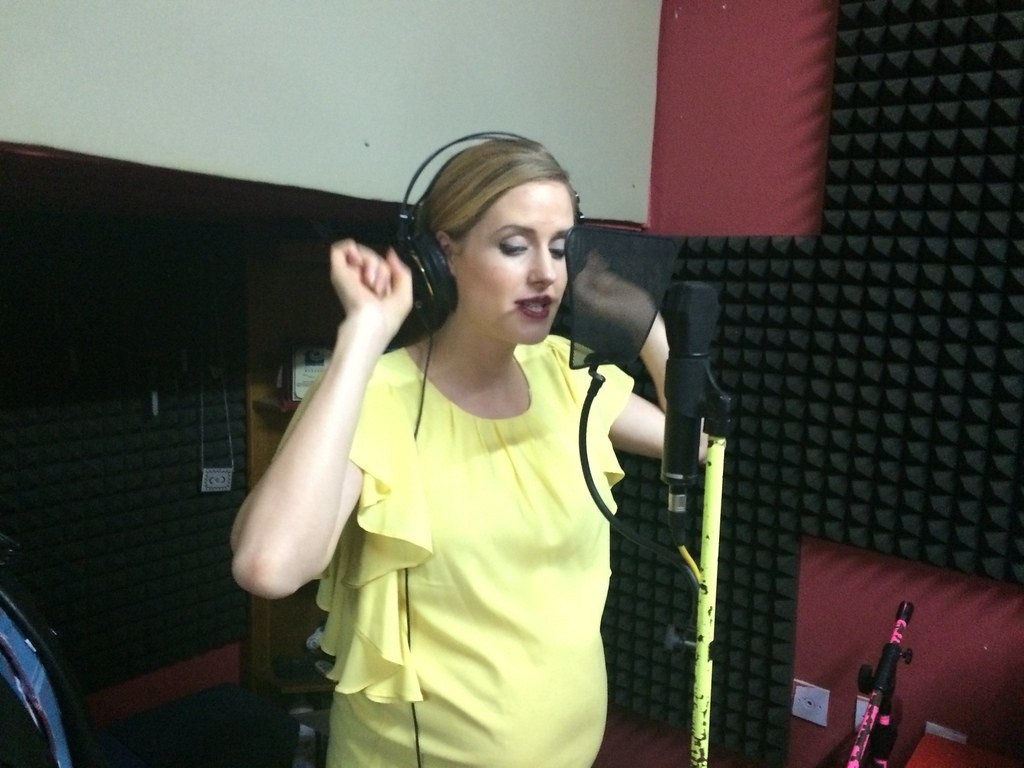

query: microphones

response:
[661,281,721,549]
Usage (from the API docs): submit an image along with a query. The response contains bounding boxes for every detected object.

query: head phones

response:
[395,130,586,333]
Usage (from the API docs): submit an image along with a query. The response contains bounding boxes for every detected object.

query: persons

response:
[231,130,706,768]
